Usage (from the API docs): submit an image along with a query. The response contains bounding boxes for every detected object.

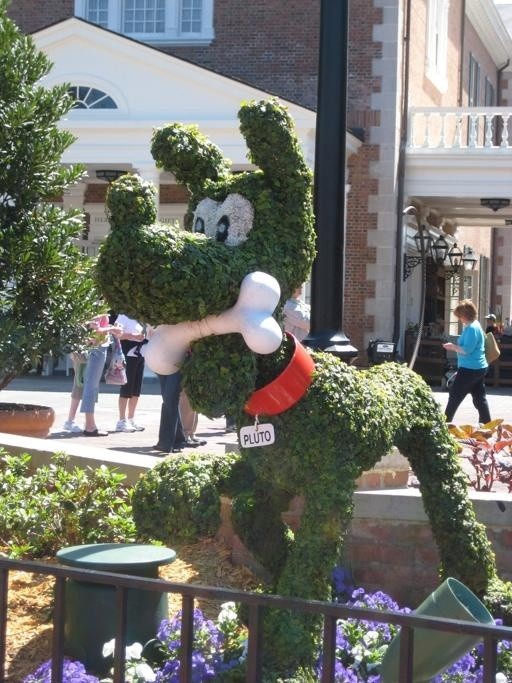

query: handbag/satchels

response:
[105,335,128,385]
[484,333,501,363]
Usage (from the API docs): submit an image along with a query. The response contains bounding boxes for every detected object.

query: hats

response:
[484,314,496,321]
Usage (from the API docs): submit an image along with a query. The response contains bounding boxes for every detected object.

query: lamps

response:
[403,225,478,281]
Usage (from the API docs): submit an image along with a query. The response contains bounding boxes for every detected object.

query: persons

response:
[79,311,123,436]
[484,313,503,335]
[440,299,491,425]
[152,370,186,453]
[62,343,98,434]
[107,309,148,432]
[179,388,207,448]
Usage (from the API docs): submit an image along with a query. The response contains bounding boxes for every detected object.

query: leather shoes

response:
[83,428,108,436]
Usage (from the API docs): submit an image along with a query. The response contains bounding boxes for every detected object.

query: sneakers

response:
[152,438,206,451]
[64,421,83,433]
[116,418,145,432]
